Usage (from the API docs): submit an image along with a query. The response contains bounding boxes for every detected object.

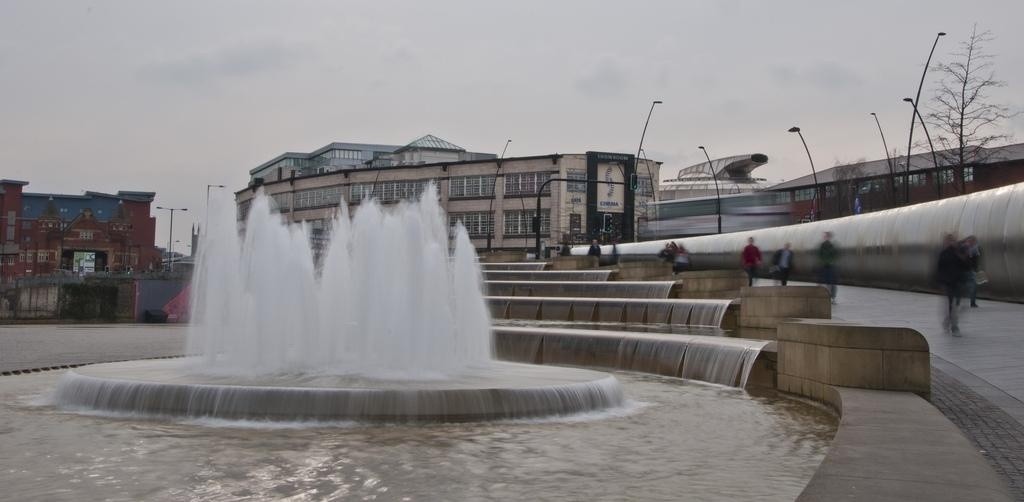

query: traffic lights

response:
[604,216,614,233]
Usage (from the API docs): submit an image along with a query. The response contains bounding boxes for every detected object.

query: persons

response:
[772,243,794,285]
[660,242,690,274]
[939,233,980,338]
[560,243,570,256]
[740,237,762,287]
[589,239,601,259]
[819,232,838,305]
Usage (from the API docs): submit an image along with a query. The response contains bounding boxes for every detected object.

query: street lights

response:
[487,138,514,254]
[167,239,181,256]
[154,205,191,271]
[205,182,225,208]
